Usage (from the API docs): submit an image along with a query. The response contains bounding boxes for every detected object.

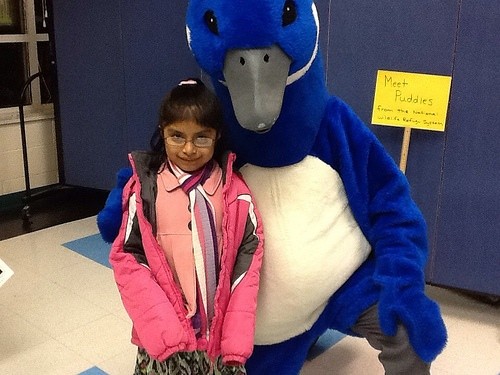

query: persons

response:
[109,79,264,375]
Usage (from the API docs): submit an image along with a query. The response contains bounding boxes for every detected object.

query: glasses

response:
[163,137,217,148]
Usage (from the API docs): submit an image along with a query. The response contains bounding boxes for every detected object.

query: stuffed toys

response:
[97,0,448,375]
